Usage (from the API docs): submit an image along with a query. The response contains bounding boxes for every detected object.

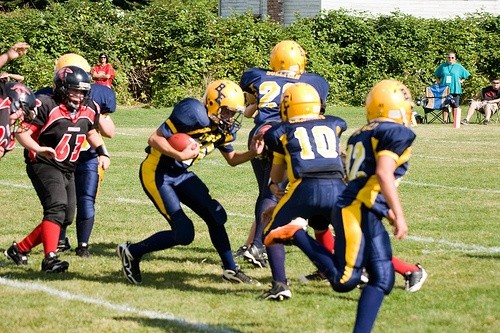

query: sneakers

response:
[118,242,143,285]
[55,238,71,253]
[42,251,69,273]
[299,269,330,283]
[265,217,309,246]
[243,243,270,270]
[460,120,469,125]
[75,244,90,258]
[483,119,490,125]
[255,280,292,302]
[4,241,29,265]
[404,264,427,293]
[222,264,261,287]
[357,269,368,289]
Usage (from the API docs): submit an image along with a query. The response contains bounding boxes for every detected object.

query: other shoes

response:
[236,245,248,257]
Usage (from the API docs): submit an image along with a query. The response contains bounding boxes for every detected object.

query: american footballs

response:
[167,133,198,153]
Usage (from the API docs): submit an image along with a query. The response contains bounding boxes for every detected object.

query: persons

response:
[262,78,417,333]
[117,80,265,287]
[434,49,471,123]
[0,42,116,275]
[234,40,426,302]
[460,78,500,125]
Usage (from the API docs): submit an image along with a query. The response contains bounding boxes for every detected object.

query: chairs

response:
[415,84,451,124]
[464,99,500,125]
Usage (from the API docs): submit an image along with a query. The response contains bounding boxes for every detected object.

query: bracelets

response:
[95,144,109,157]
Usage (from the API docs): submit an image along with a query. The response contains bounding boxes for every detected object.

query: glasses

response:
[100,57,106,58]
[448,57,454,59]
[493,82,499,84]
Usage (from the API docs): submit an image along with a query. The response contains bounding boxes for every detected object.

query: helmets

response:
[4,81,36,133]
[203,79,245,136]
[281,83,321,122]
[366,80,413,128]
[270,40,306,75]
[54,53,92,111]
